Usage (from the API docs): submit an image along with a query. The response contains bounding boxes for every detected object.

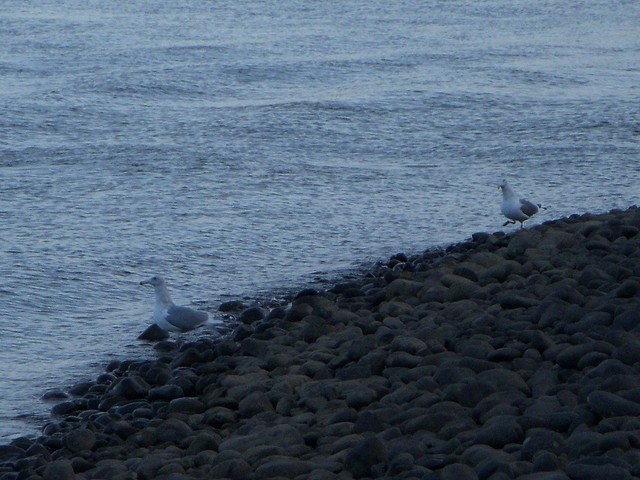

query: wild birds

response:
[497,178,539,230]
[140,275,209,333]
